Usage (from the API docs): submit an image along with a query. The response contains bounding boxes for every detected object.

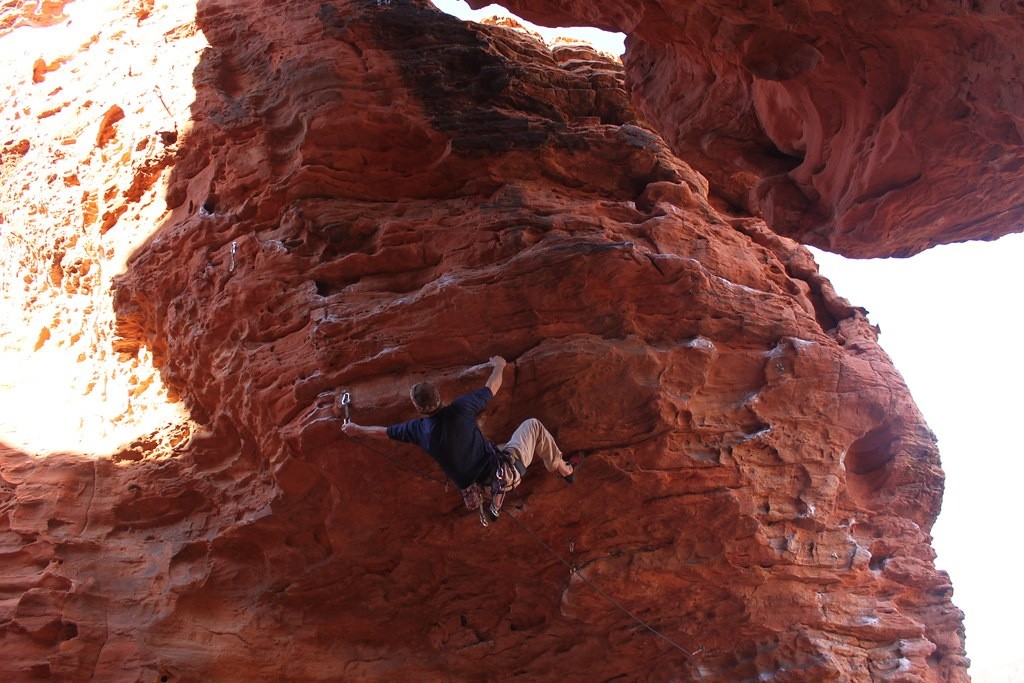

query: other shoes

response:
[563,459,579,484]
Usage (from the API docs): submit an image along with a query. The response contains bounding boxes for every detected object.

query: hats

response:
[408,380,441,414]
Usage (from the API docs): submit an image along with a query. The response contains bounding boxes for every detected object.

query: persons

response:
[342,356,585,521]
